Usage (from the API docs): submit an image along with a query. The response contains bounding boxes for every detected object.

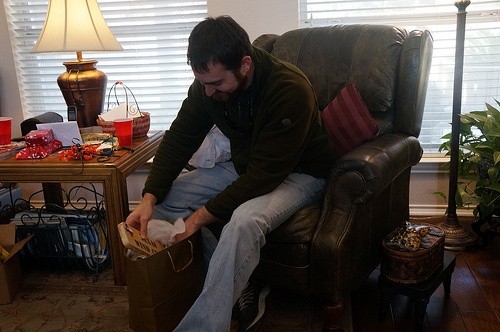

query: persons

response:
[125,16,335,332]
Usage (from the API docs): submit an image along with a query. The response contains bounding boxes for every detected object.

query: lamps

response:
[29,0,125,129]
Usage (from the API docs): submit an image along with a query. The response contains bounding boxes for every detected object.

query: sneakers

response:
[236,283,270,332]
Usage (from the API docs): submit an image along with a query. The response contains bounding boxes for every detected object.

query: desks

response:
[0,129,168,287]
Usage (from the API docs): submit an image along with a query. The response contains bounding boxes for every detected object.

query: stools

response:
[369,253,457,332]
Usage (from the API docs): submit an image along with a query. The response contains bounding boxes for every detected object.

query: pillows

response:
[318,82,382,156]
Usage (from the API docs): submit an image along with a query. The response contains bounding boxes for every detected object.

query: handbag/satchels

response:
[127,227,201,332]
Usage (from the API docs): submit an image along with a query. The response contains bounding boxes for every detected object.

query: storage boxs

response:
[0,223,35,305]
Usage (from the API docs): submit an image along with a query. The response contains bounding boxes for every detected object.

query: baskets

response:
[96,81,150,139]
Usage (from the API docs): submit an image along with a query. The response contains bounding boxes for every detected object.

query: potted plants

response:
[424,97,500,235]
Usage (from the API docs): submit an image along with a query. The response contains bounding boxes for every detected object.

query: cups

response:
[0,117,14,150]
[113,118,134,146]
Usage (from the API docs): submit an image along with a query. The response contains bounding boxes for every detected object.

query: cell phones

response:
[67,106,77,122]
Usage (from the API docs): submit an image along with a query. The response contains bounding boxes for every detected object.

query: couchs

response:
[205,25,433,332]
